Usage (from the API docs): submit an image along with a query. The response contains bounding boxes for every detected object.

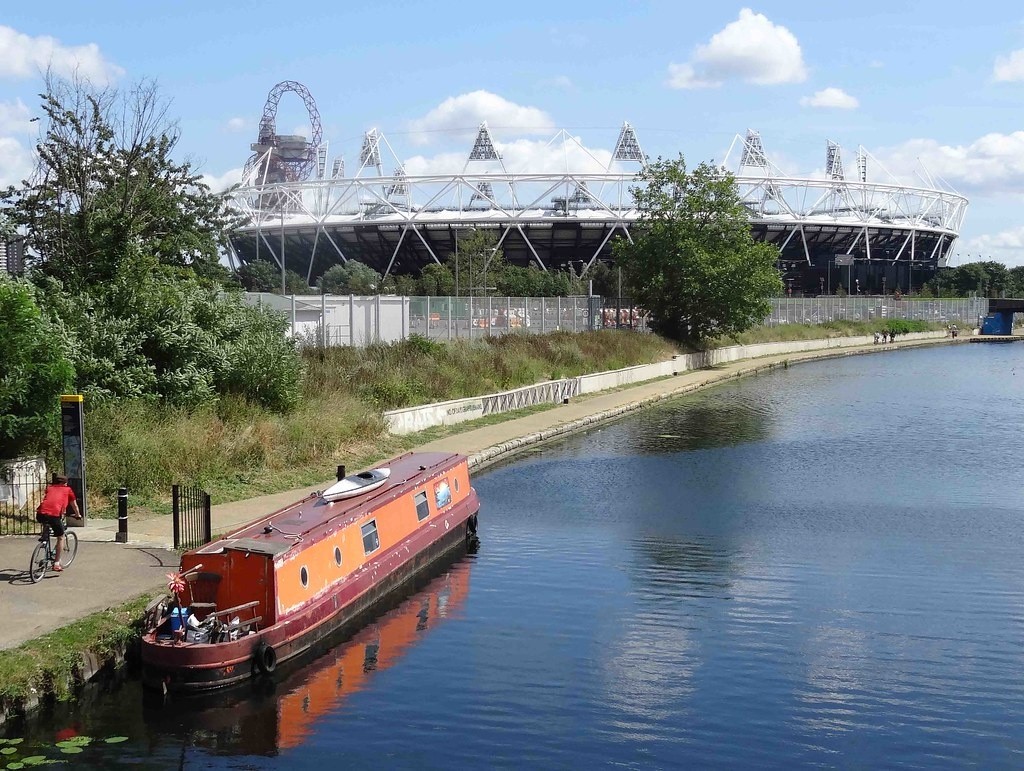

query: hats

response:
[56,476,69,483]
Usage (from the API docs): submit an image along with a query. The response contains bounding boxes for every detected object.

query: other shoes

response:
[52,564,64,571]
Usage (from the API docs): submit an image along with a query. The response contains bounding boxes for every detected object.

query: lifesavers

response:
[256,644,276,673]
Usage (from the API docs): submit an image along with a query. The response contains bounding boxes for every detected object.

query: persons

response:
[874,329,895,344]
[951,324,956,338]
[36,476,81,571]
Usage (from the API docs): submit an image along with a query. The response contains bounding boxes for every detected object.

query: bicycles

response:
[29,514,82,584]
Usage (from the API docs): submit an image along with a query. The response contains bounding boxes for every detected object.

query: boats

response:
[145,557,471,759]
[136,449,480,695]
[322,467,391,502]
